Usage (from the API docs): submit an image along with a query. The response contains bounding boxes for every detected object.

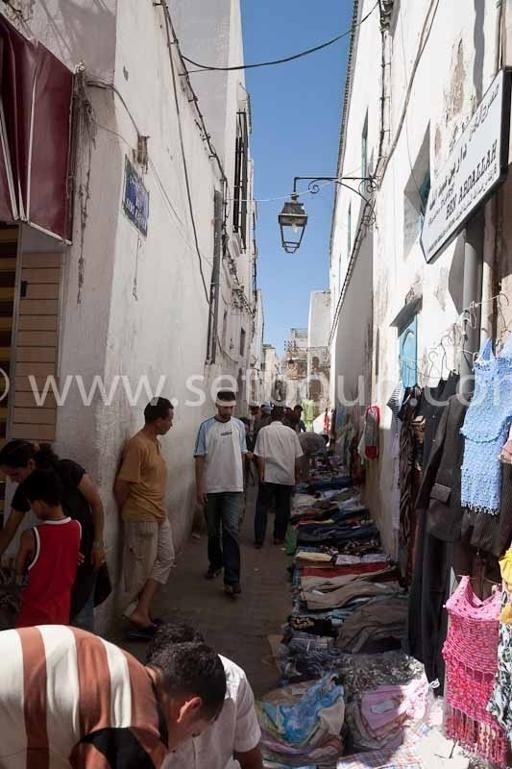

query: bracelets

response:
[91,541,105,550]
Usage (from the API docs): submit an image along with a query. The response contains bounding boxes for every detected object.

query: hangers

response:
[413,293,512,393]
[451,547,503,596]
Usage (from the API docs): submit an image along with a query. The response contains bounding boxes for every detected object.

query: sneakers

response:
[224,584,242,596]
[203,566,222,579]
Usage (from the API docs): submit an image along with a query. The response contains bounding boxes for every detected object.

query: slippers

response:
[126,621,158,641]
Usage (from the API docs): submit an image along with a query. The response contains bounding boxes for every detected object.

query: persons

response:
[10,471,87,629]
[239,400,329,550]
[146,620,265,769]
[110,396,175,643]
[0,622,227,768]
[0,436,112,634]
[194,389,249,596]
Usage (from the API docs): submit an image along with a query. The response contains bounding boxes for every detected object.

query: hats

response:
[215,390,237,409]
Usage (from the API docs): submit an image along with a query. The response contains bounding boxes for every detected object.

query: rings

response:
[101,558,105,565]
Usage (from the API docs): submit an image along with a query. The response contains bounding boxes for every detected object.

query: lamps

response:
[287,346,308,369]
[279,175,376,253]
